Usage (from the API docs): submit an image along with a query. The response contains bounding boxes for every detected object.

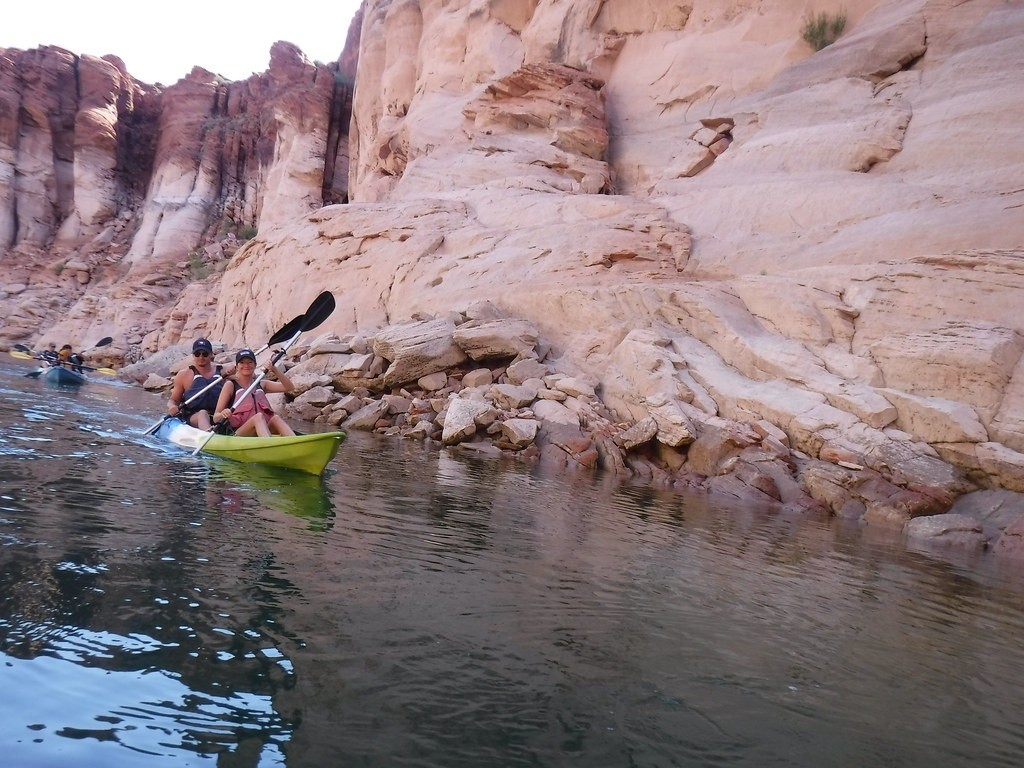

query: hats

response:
[236,348,256,364]
[191,337,213,354]
[49,342,56,347]
[59,343,72,351]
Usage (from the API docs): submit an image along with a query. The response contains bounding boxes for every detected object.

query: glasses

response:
[194,351,211,357]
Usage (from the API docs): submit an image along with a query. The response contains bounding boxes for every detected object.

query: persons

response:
[167,338,236,432]
[212,349,297,438]
[36,342,83,372]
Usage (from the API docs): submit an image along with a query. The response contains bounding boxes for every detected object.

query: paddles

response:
[10,351,117,377]
[143,313,306,437]
[25,336,113,379]
[191,291,337,459]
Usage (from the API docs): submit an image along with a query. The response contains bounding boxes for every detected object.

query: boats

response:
[9,350,35,360]
[41,360,54,368]
[154,411,347,477]
[36,365,87,387]
